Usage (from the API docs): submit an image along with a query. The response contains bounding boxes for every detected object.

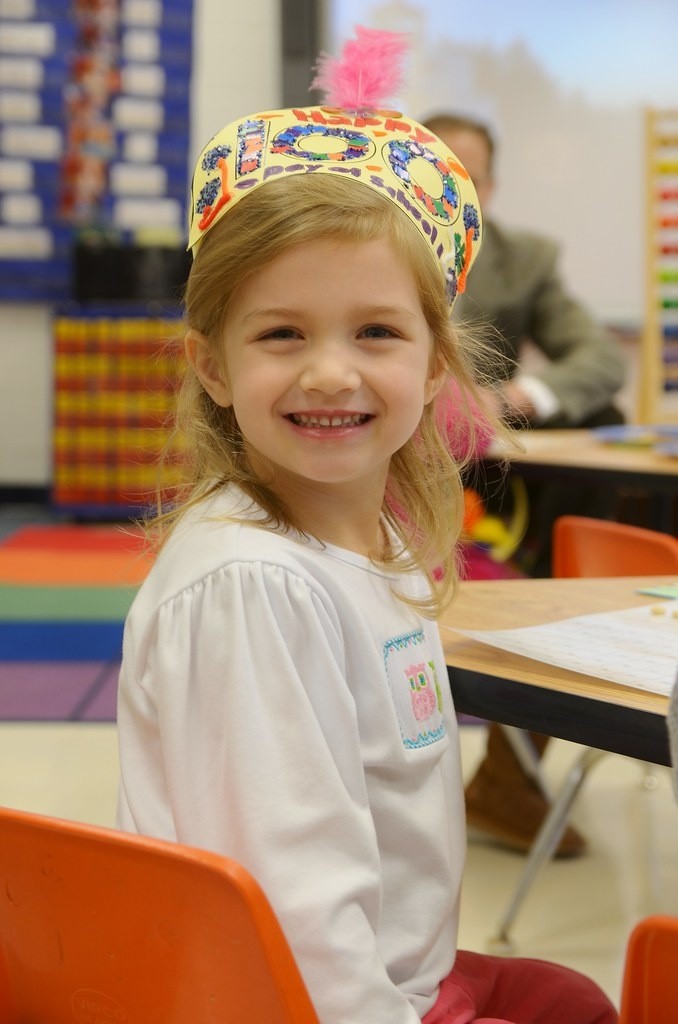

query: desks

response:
[460,424,677,494]
[408,574,677,768]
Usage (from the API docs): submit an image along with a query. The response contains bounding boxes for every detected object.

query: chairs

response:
[0,807,321,1024]
[499,516,678,947]
[617,916,678,1024]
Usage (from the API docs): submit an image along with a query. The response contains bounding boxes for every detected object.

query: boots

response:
[465,723,587,858]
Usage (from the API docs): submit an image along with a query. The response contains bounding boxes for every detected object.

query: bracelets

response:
[489,379,511,420]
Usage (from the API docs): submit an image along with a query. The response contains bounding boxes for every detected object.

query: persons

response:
[114,103,618,1024]
[406,115,626,856]
